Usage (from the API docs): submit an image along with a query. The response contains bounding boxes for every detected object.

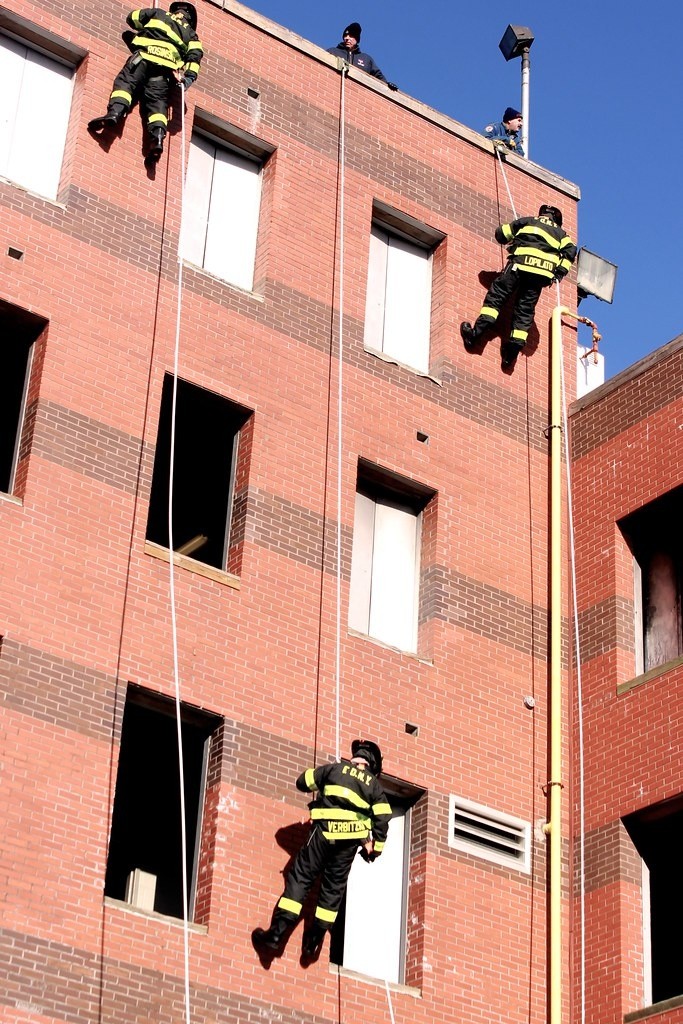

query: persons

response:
[459,205,577,369]
[482,107,525,156]
[327,23,399,89]
[88,2,203,153]
[253,740,392,964]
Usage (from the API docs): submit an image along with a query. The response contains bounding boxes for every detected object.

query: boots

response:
[252,917,295,950]
[302,926,327,963]
[150,127,164,154]
[88,109,120,131]
[501,342,522,369]
[461,322,481,352]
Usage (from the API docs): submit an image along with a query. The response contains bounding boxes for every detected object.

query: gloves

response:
[495,139,506,147]
[553,271,563,283]
[369,851,381,863]
[177,77,192,91]
[510,139,516,149]
[386,82,399,89]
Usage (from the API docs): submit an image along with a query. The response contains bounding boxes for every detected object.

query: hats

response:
[343,22,362,43]
[503,107,523,122]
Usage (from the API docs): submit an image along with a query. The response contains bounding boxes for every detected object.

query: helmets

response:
[352,740,382,773]
[170,2,197,30]
[538,205,562,227]
